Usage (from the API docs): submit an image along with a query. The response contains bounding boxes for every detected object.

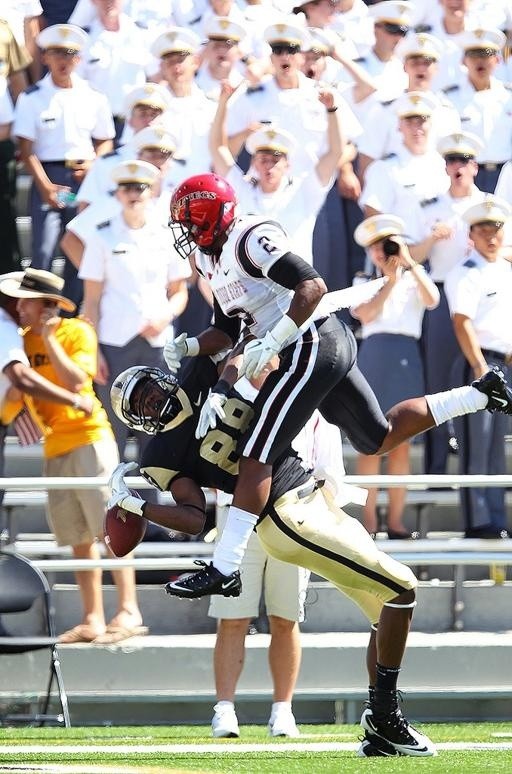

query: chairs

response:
[1,549,75,726]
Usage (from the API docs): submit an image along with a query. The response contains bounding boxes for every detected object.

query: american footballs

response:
[104,489,147,557]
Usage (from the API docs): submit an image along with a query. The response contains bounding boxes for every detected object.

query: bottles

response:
[123,437,139,476]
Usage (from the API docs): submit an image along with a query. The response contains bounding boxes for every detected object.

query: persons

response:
[207,410,344,735]
[0,0,512,644]
[107,338,436,755]
[164,173,512,600]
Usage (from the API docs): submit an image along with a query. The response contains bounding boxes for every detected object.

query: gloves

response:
[195,392,228,439]
[237,330,280,378]
[164,334,187,374]
[106,462,139,510]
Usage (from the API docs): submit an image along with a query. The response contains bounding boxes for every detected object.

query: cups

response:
[490,560,507,586]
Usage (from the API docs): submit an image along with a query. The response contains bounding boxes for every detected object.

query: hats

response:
[1,269,78,314]
[368,1,415,35]
[435,130,484,159]
[461,200,512,227]
[354,214,407,248]
[35,23,89,56]
[112,160,160,191]
[398,33,446,62]
[245,127,295,159]
[264,23,307,53]
[304,27,335,56]
[148,27,199,61]
[458,25,507,56]
[389,91,441,121]
[206,17,247,44]
[131,125,179,155]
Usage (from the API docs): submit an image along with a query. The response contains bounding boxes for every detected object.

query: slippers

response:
[57,622,151,645]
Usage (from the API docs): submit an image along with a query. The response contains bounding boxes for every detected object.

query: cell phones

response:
[44,299,57,308]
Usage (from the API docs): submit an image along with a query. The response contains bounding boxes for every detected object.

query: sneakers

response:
[472,367,511,415]
[269,713,298,738]
[361,704,437,758]
[164,562,242,598]
[211,709,240,737]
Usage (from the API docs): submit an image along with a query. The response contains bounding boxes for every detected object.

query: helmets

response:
[170,172,239,256]
[110,367,182,437]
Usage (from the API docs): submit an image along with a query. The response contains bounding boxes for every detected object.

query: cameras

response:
[383,239,399,260]
